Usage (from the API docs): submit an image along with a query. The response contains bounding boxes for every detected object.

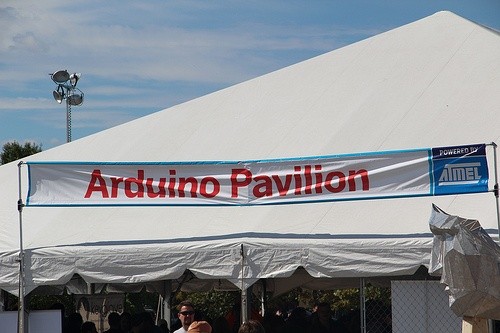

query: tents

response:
[0,11,500,333]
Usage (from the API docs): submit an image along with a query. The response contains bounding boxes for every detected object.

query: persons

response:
[212,298,361,333]
[188,320,212,333]
[172,301,194,333]
[51,300,170,333]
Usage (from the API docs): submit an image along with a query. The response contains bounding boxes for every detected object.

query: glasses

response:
[180,311,195,315]
